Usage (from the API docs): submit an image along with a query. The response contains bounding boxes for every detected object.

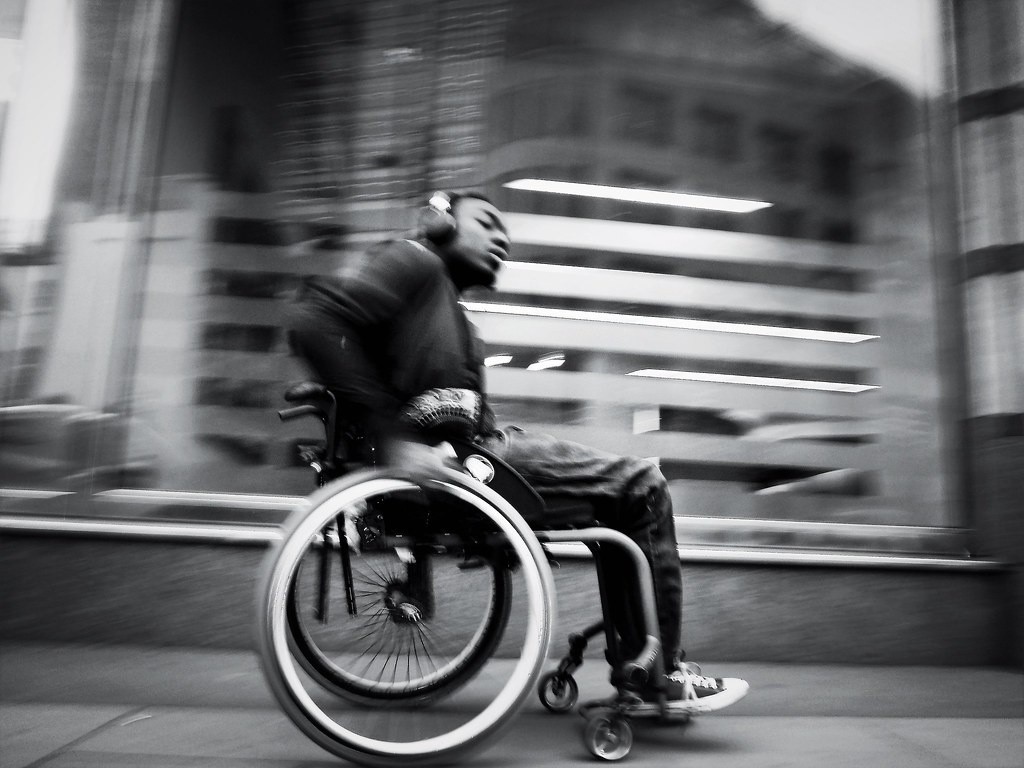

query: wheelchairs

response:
[249,377,698,768]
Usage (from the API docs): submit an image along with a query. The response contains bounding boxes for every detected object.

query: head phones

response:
[422,190,458,243]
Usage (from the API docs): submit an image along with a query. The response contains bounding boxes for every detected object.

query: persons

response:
[284,190,749,718]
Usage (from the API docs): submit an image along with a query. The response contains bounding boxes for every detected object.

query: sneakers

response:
[618,652,750,716]
